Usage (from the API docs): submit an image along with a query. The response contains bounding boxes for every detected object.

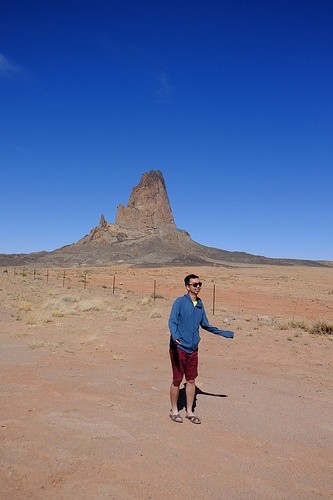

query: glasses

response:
[186,282,202,287]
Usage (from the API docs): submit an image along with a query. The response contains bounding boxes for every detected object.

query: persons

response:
[168,274,235,425]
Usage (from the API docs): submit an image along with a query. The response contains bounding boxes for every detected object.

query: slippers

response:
[169,413,183,424]
[186,415,201,424]
